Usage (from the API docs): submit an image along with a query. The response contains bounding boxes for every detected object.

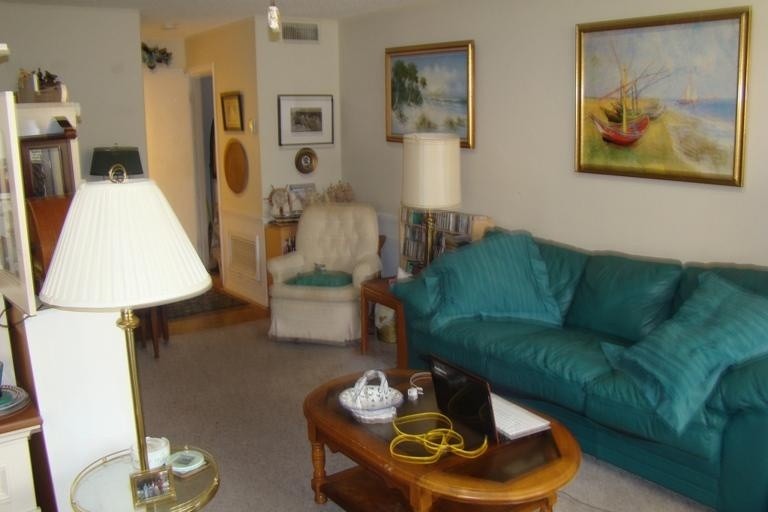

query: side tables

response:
[354,276,423,366]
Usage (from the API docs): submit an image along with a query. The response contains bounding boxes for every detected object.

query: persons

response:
[30,160,50,197]
[140,479,169,498]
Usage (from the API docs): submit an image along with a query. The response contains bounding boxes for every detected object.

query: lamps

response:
[90,145,143,179]
[400,134,459,277]
[37,166,218,511]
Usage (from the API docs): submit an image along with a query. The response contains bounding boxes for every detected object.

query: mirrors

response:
[22,135,79,199]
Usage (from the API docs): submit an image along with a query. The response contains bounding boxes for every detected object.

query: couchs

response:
[386,231,768,511]
[265,199,384,347]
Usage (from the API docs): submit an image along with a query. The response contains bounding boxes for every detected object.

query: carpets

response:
[132,318,717,512]
[167,290,245,318]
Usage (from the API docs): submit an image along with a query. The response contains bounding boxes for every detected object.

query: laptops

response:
[426,352,551,446]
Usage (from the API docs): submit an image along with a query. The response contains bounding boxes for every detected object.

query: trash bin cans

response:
[373,303,396,345]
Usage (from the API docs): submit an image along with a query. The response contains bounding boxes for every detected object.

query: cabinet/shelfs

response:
[266,221,296,307]
[397,206,491,277]
[394,203,488,287]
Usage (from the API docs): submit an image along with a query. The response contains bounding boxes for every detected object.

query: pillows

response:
[284,269,351,287]
[601,275,768,430]
[412,228,560,335]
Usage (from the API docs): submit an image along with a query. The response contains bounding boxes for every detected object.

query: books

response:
[431,232,468,260]
[401,225,427,261]
[400,206,474,235]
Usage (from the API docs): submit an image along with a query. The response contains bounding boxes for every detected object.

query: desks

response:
[358,277,409,369]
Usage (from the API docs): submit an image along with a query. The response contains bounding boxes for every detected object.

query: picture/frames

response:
[129,466,177,506]
[573,6,751,189]
[277,92,334,150]
[286,183,320,213]
[384,39,479,150]
[219,90,246,133]
